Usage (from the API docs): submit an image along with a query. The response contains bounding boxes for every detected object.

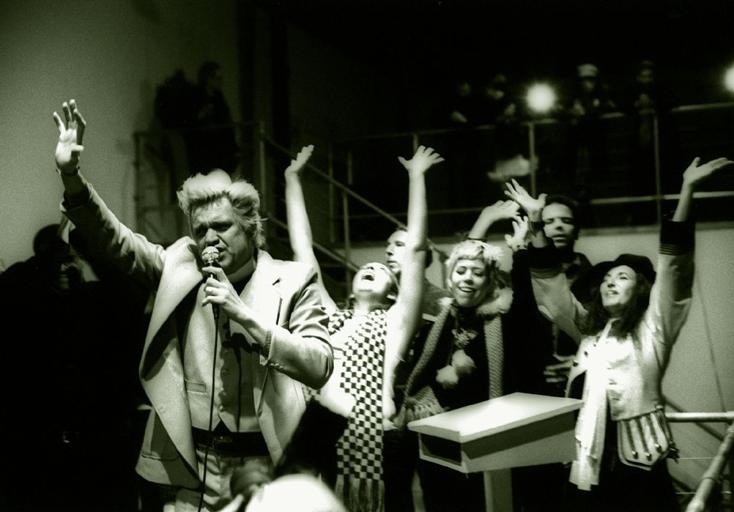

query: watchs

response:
[526,221,551,235]
[54,165,82,183]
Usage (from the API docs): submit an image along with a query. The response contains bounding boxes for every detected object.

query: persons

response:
[192,61,240,178]
[50,98,334,512]
[467,198,601,512]
[386,226,454,512]
[504,157,734,512]
[284,144,445,512]
[392,216,550,512]
[0,223,139,512]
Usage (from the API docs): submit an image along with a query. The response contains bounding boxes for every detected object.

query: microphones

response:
[201,248,225,321]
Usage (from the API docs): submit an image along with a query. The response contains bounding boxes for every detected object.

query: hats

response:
[586,253,655,290]
